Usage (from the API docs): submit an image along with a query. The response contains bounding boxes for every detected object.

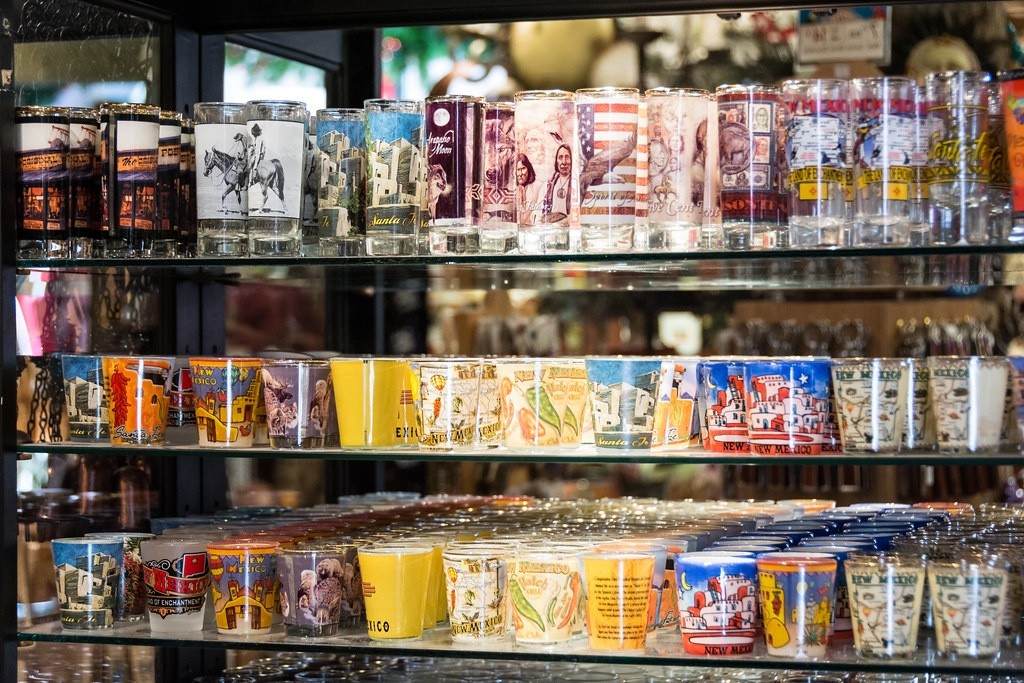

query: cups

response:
[18,352,1024,458]
[13,70,1023,252]
[18,639,1024,683]
[52,491,1024,671]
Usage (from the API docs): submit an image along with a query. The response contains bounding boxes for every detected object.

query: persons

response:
[515,127,572,227]
[233,123,266,191]
